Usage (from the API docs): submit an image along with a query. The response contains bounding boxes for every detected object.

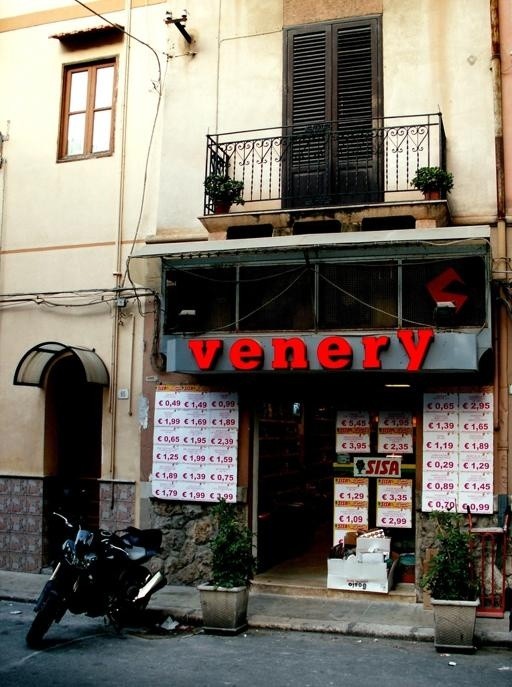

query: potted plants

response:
[410,167,453,200]
[205,175,245,214]
[198,496,255,637]
[429,506,484,655]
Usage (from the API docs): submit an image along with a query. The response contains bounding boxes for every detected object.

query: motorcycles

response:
[25,512,168,649]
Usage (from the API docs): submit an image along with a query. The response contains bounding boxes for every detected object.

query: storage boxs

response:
[325,531,399,594]
